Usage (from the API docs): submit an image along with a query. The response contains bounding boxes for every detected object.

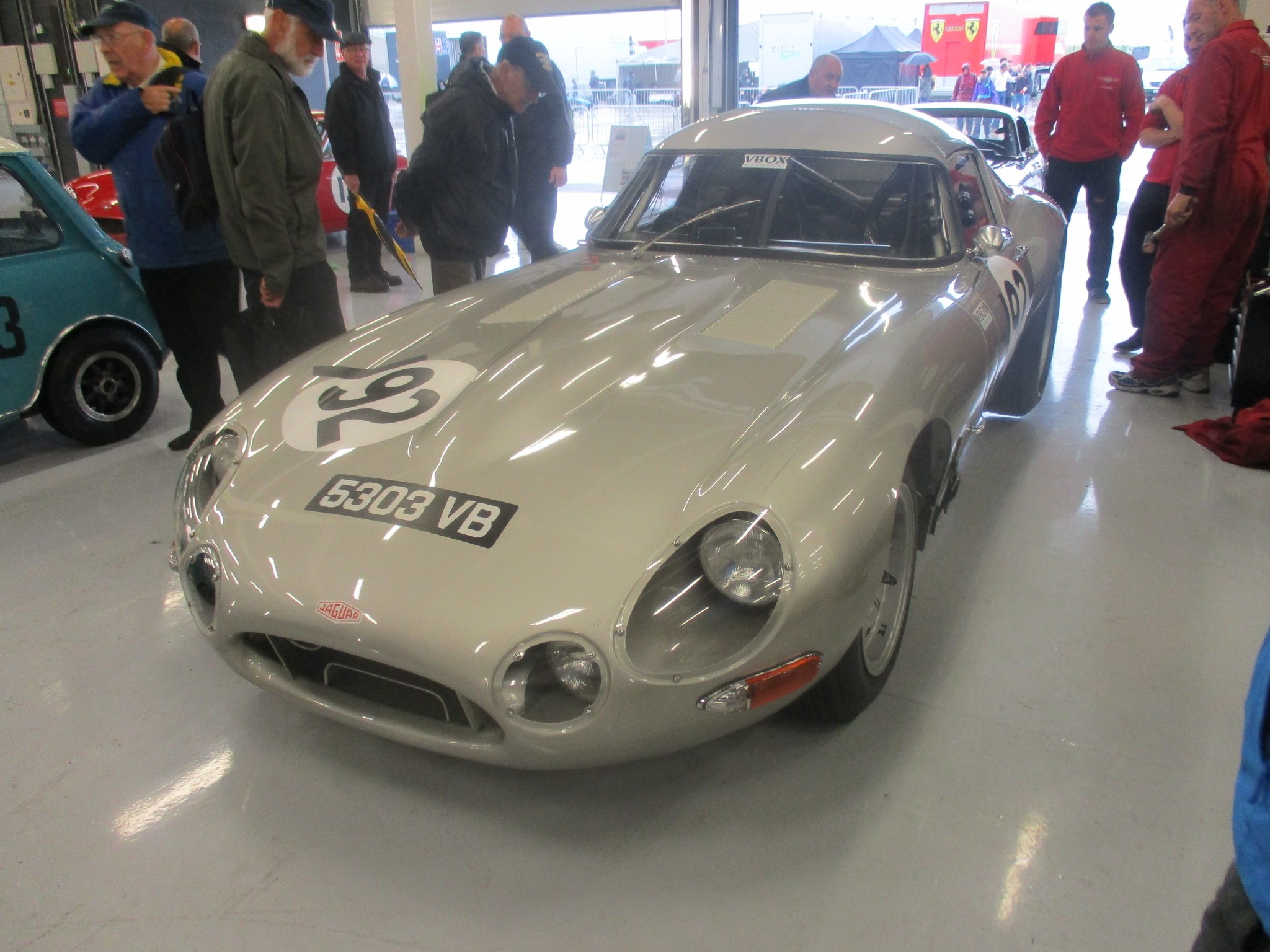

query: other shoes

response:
[167,395,226,451]
[372,266,403,287]
[1173,366,1211,394]
[1088,286,1110,304]
[1108,369,1180,397]
[349,274,392,293]
[1114,327,1144,353]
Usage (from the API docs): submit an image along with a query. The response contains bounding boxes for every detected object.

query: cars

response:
[61,111,410,247]
[1140,66,1180,102]
[903,101,1048,193]
[0,136,170,444]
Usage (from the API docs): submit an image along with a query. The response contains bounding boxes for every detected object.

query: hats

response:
[340,31,372,49]
[269,0,343,44]
[80,1,159,38]
[497,36,560,96]
[459,30,480,52]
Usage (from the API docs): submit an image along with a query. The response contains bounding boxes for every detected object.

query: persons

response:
[1034,2,1146,303]
[161,18,202,73]
[1108,0,1270,398]
[324,33,402,293]
[674,67,681,107]
[624,71,638,113]
[572,69,600,106]
[392,35,559,296]
[922,63,935,90]
[459,15,575,264]
[738,69,754,104]
[70,2,240,450]
[950,58,1033,140]
[757,54,843,103]
[1190,627,1270,952]
[204,0,348,379]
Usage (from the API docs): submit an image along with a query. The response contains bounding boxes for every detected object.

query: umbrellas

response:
[902,53,938,66]
[980,57,1000,67]
[354,193,424,291]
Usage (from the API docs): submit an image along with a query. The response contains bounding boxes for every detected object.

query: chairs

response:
[857,163,931,257]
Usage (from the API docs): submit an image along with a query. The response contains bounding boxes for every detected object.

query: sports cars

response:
[164,97,1068,774]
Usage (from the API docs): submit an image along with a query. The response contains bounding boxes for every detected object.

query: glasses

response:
[96,30,141,46]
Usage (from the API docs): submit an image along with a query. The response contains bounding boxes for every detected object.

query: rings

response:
[1178,218,1182,223]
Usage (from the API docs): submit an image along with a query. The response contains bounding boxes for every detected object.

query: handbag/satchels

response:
[152,88,217,230]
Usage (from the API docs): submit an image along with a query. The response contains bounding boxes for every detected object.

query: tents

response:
[616,23,921,105]
